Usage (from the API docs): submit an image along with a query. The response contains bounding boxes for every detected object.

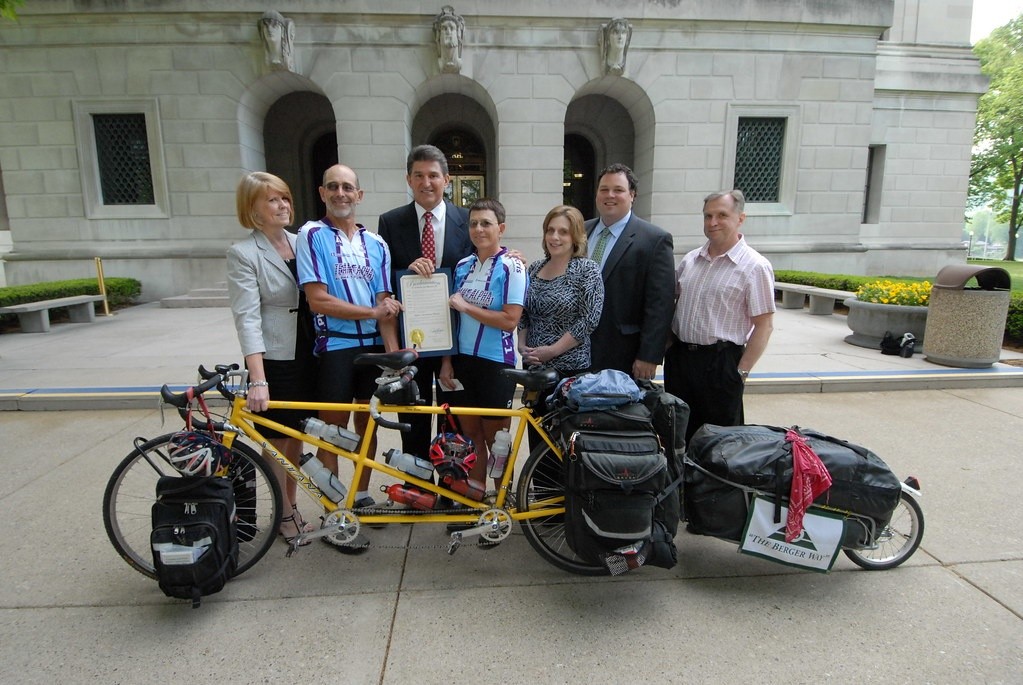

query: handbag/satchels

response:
[679,424,902,549]
[647,518,679,570]
[544,368,652,431]
[880,330,918,357]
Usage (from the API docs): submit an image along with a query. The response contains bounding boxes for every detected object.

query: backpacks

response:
[151,476,239,610]
[562,427,667,566]
[634,377,691,523]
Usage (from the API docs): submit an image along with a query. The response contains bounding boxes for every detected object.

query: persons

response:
[605,17,629,68]
[295,164,405,556]
[437,15,462,73]
[378,145,527,525]
[664,189,776,536]
[517,205,606,528]
[257,10,286,65]
[224,171,317,547]
[583,163,675,380]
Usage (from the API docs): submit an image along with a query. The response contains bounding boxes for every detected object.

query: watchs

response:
[738,369,748,377]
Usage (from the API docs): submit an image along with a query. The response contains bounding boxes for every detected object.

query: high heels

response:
[278,504,314,546]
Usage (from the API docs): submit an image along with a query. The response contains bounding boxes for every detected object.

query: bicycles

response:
[103,347,650,581]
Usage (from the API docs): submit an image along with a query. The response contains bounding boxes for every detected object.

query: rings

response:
[416,263,419,266]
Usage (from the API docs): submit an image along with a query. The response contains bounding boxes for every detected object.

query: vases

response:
[844,297,928,355]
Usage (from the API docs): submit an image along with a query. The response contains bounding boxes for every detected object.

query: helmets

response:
[167,431,232,477]
[429,432,477,481]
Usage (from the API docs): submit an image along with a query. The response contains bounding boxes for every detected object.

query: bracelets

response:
[248,381,268,387]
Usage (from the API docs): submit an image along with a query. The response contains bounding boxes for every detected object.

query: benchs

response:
[774,279,857,316]
[0,294,106,335]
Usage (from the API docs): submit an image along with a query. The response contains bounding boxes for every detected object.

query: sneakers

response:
[319,515,372,555]
[352,497,389,529]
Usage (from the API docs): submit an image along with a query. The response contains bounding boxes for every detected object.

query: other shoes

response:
[447,499,494,535]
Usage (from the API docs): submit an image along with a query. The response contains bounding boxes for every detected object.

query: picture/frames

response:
[457,175,485,209]
[444,176,457,206]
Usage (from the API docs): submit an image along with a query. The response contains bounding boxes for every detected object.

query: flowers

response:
[856,279,933,307]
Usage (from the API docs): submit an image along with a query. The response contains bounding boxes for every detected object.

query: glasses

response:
[323,182,360,192]
[468,219,498,226]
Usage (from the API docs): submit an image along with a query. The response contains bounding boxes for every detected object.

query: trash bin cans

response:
[922,262,1012,369]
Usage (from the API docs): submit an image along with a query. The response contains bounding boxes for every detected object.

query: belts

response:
[671,337,735,351]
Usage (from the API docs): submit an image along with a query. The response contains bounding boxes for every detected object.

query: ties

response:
[422,211,436,271]
[590,228,610,264]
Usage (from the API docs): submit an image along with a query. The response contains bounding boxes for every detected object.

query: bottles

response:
[300,416,361,452]
[443,475,485,502]
[380,483,436,511]
[298,452,348,504]
[488,428,512,479]
[382,449,434,480]
